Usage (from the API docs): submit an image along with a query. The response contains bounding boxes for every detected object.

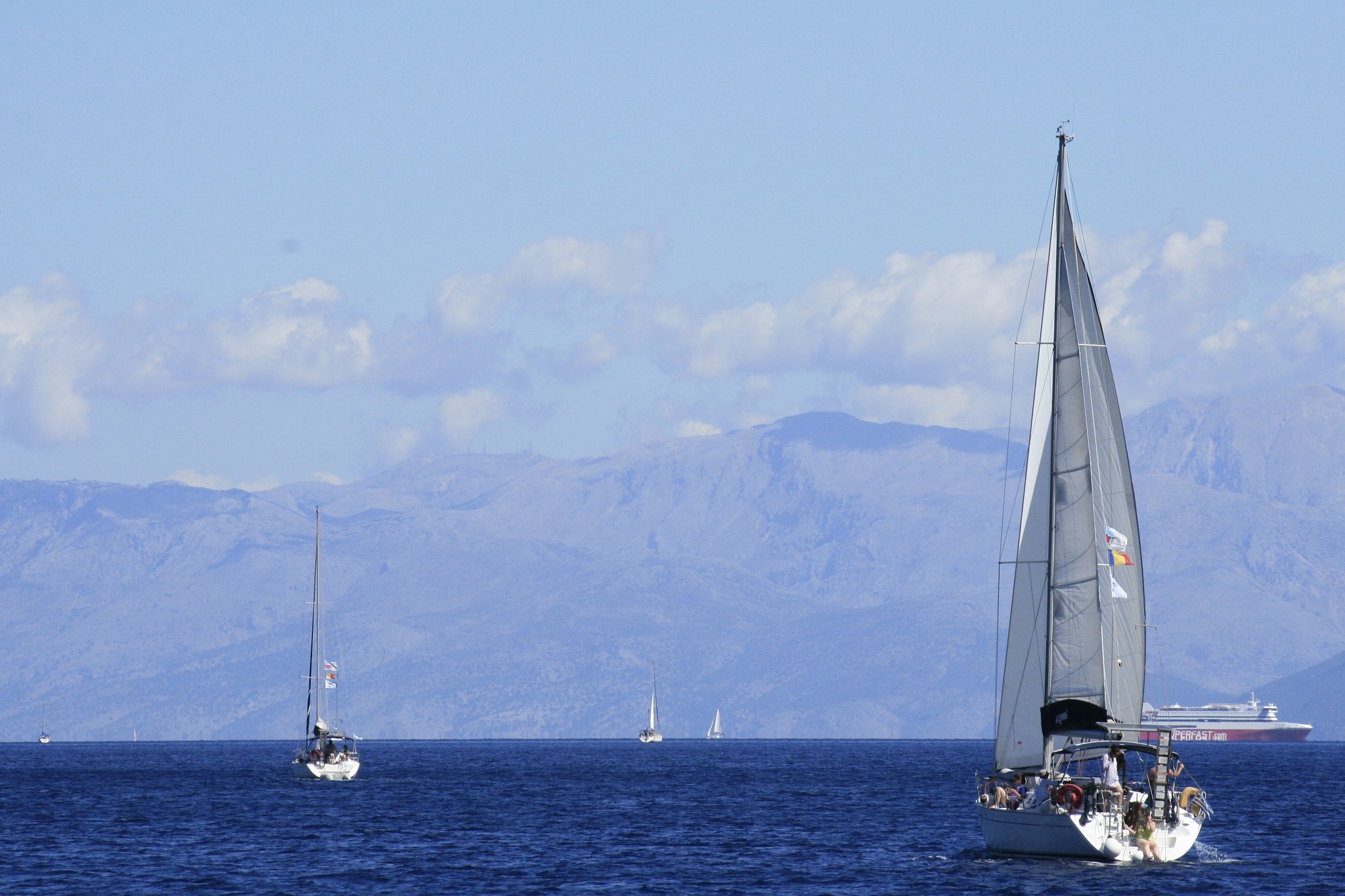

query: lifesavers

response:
[1179,786,1200,814]
[1057,784,1084,809]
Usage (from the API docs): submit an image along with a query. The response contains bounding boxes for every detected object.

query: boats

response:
[1140,692,1313,741]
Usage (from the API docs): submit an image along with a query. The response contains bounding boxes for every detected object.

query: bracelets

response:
[1149,822,1151,823]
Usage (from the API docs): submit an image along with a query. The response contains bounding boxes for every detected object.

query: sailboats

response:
[39,695,50,743]
[975,120,1214,861]
[330,674,343,741]
[639,654,662,743]
[707,708,724,739]
[292,505,363,781]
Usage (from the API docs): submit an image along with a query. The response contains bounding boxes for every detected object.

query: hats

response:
[1039,769,1050,778]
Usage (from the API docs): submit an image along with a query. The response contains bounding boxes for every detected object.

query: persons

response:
[1146,763,1184,805]
[1035,769,1058,806]
[1100,747,1126,815]
[326,740,336,759]
[341,745,349,759]
[1123,806,1167,862]
[987,773,1025,809]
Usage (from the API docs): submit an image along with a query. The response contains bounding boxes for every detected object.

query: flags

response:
[325,680,336,688]
[325,660,338,671]
[325,672,337,680]
[1112,578,1128,599]
[1107,549,1134,566]
[1106,526,1128,552]
